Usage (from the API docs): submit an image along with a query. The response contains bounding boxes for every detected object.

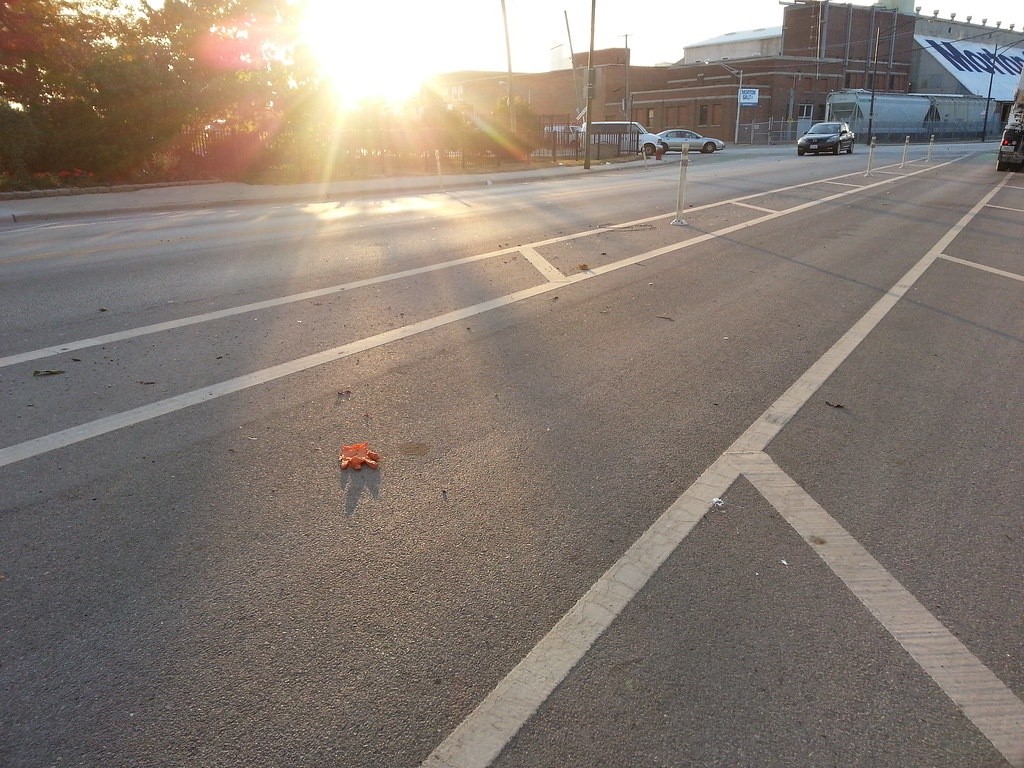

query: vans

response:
[543,126,583,147]
[581,122,662,156]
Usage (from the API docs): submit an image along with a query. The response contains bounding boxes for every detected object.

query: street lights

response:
[867,17,934,146]
[704,58,743,144]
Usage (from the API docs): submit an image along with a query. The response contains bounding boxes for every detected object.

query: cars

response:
[656,129,725,153]
[797,122,855,155]
